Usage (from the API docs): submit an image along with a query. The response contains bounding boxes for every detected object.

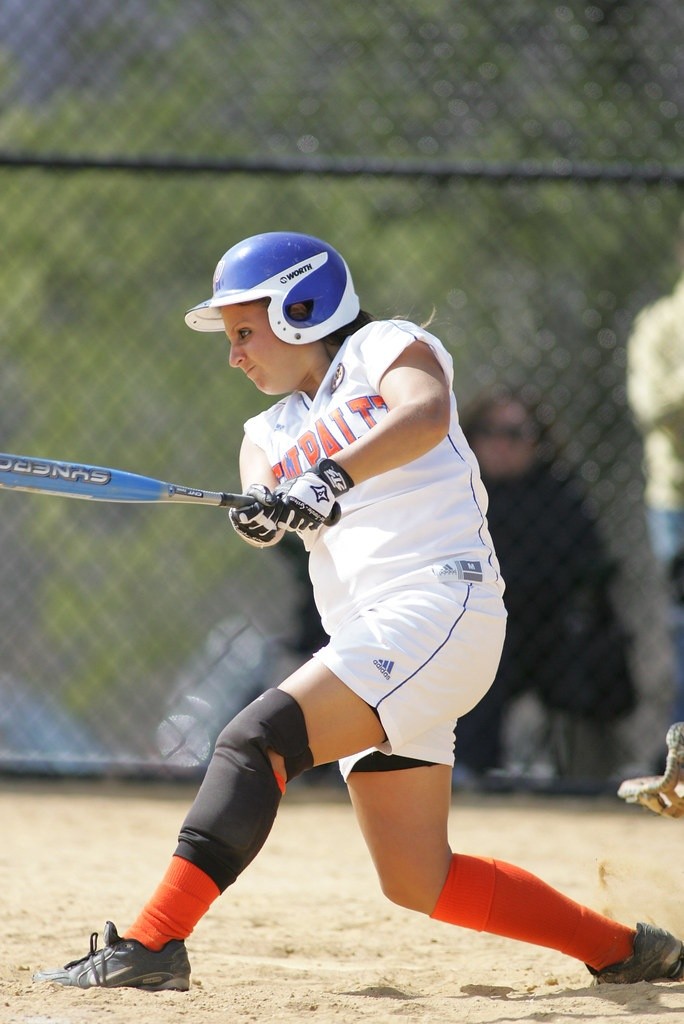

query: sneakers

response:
[32,921,191,992]
[585,922,684,988]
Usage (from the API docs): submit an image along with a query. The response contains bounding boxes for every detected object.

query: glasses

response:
[476,422,522,440]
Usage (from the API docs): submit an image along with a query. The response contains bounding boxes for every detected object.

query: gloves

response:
[228,484,285,547]
[273,458,354,535]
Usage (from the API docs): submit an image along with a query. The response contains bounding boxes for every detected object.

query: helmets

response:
[185,231,360,345]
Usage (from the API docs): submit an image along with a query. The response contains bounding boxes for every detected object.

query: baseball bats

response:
[0,449,345,532]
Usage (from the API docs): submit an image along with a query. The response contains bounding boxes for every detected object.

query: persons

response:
[624,276,684,777]
[454,383,639,793]
[43,232,684,991]
